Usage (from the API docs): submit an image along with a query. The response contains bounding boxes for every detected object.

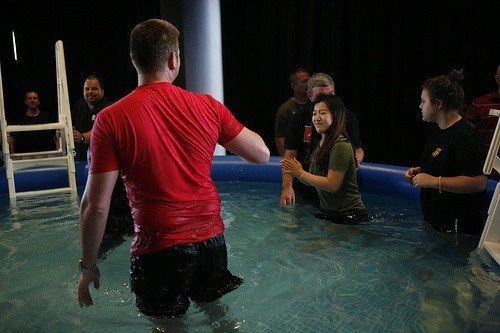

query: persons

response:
[280,73,364,206]
[275,69,310,156]
[9,91,61,153]
[77,19,270,316]
[54,76,113,161]
[281,94,367,222]
[404,70,488,236]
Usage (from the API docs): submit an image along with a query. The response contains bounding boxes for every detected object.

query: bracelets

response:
[437,175,445,195]
[81,133,86,144]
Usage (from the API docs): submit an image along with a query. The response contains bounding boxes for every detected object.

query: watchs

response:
[78,257,98,273]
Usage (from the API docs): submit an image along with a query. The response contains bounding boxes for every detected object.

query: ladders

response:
[477,114,500,267]
[0,41,78,229]
